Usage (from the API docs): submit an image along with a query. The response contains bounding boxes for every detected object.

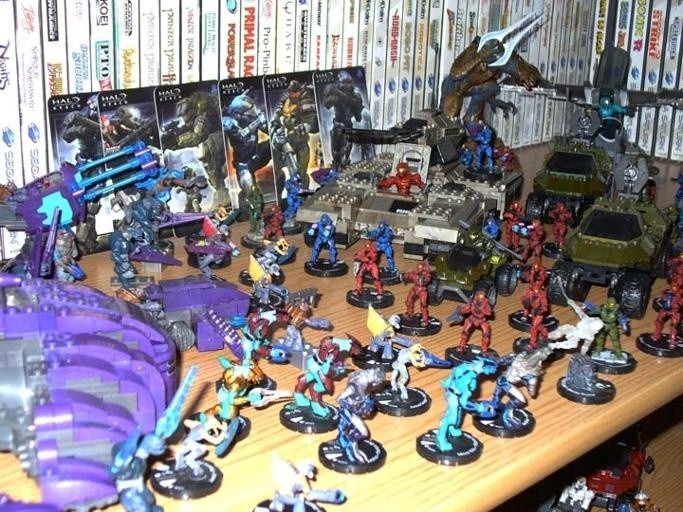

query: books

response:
[0,0,683,268]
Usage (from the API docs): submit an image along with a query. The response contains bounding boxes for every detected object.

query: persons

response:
[215,310,293,407]
[415,355,499,465]
[584,294,638,375]
[184,352,263,444]
[243,236,291,316]
[393,258,442,337]
[507,259,560,333]
[346,240,395,309]
[150,412,231,500]
[501,197,533,268]
[635,250,683,359]
[351,304,413,373]
[178,215,242,288]
[517,217,555,286]
[464,120,504,182]
[263,170,305,235]
[554,275,618,406]
[420,221,518,307]
[252,205,297,266]
[362,219,405,287]
[103,190,210,293]
[370,342,453,418]
[262,289,334,365]
[513,276,566,364]
[444,288,498,369]
[539,200,574,261]
[471,344,553,440]
[306,213,350,279]
[252,458,346,512]
[111,364,199,512]
[278,333,353,435]
[296,10,550,256]
[318,366,387,475]
